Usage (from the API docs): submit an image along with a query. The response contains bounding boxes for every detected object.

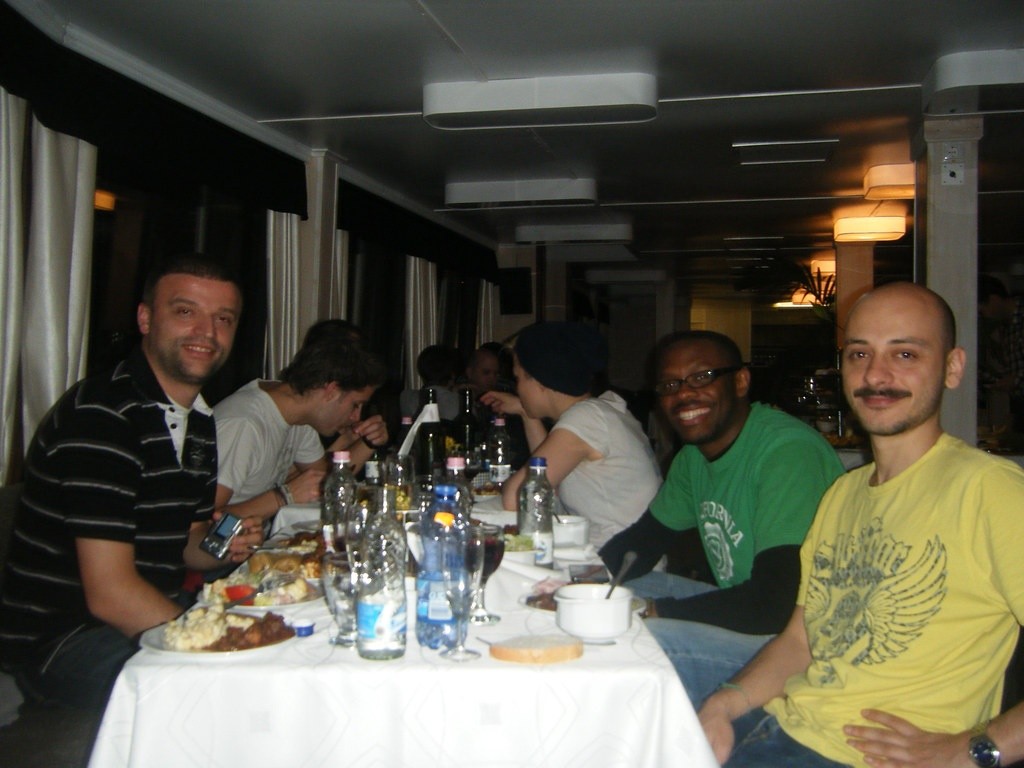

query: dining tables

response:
[271,475,518,535]
[88,521,723,768]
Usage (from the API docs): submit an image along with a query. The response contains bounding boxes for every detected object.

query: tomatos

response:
[226,584,261,606]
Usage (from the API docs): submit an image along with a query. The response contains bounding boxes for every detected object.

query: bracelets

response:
[718,682,752,714]
[275,484,291,506]
[362,436,380,450]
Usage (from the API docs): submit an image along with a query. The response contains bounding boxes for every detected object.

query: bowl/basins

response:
[552,514,590,548]
[552,582,634,645]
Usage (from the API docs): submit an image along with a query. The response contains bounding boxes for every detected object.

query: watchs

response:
[968,734,1000,768]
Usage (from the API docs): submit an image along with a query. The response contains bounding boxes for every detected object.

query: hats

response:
[514,322,608,397]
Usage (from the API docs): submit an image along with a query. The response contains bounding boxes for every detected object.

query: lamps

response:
[791,293,829,306]
[834,216,906,242]
[585,270,666,285]
[515,224,633,244]
[919,47,1024,117]
[810,261,837,279]
[864,164,917,199]
[423,73,660,129]
[446,179,601,212]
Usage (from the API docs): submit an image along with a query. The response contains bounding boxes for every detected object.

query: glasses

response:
[650,367,735,395]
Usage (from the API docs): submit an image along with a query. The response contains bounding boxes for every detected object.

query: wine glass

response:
[441,524,485,662]
[468,523,505,626]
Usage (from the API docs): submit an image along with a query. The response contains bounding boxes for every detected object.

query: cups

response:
[321,550,365,649]
[382,453,416,523]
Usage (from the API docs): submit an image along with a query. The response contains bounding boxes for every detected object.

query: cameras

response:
[197,511,245,561]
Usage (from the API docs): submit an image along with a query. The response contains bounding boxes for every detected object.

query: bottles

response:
[516,457,554,570]
[320,386,516,663]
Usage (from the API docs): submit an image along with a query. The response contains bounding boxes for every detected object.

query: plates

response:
[138,610,305,662]
[196,576,327,610]
[239,548,322,582]
[517,592,647,613]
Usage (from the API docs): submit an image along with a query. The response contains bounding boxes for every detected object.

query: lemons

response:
[434,511,455,527]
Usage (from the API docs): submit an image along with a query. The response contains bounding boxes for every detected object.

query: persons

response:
[694,282,1024,768]
[600,328,847,713]
[176,318,662,616]
[0,249,263,768]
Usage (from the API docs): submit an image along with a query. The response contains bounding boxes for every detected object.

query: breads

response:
[491,633,583,664]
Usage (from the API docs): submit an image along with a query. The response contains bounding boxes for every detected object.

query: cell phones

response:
[569,564,609,583]
